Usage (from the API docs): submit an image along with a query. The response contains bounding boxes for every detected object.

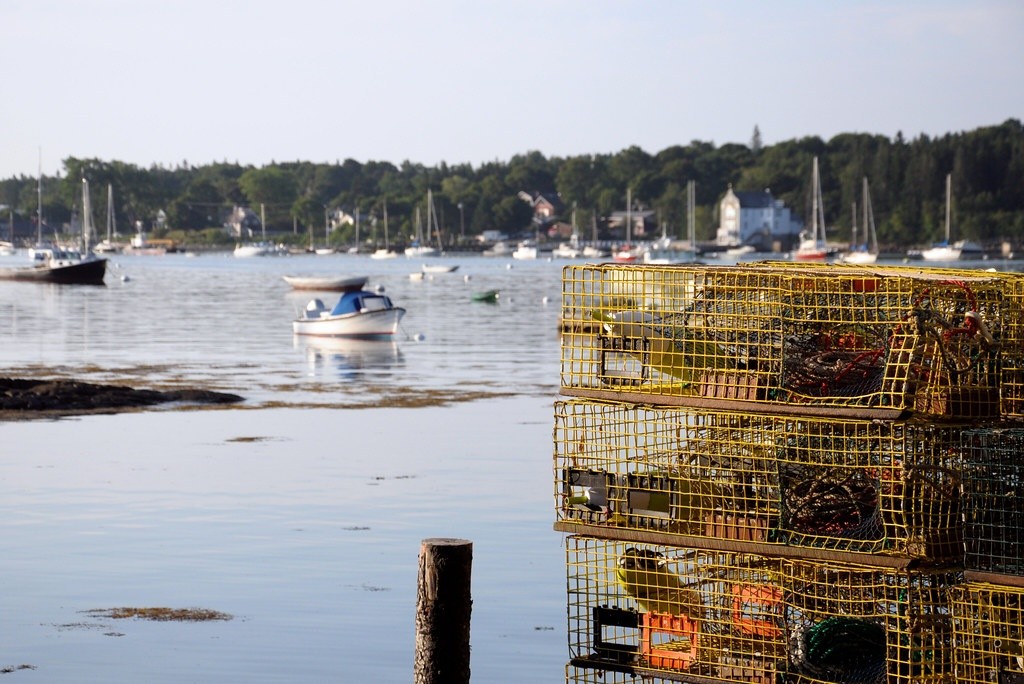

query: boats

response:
[511,154,964,267]
[421,265,458,275]
[234,247,266,257]
[370,250,398,260]
[293,291,405,337]
[282,272,367,294]
[470,289,497,301]
[403,245,440,256]
[0,259,108,284]
